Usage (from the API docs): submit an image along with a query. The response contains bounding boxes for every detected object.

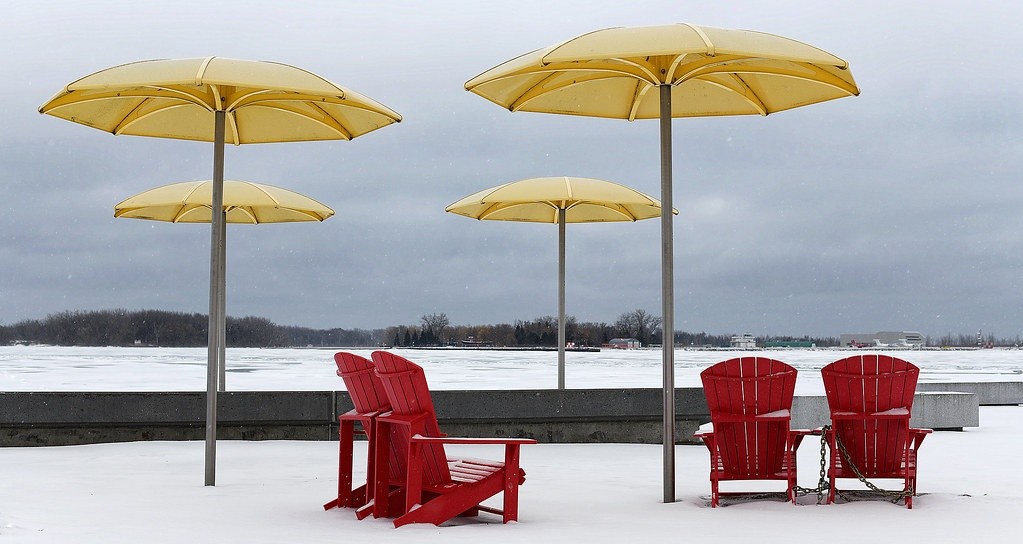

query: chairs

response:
[323,351,538,527]
[693,356,814,507]
[812,353,934,510]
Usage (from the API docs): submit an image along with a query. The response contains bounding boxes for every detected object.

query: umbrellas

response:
[114,182,333,391]
[446,175,679,384]
[466,25,860,502]
[39,54,402,486]
